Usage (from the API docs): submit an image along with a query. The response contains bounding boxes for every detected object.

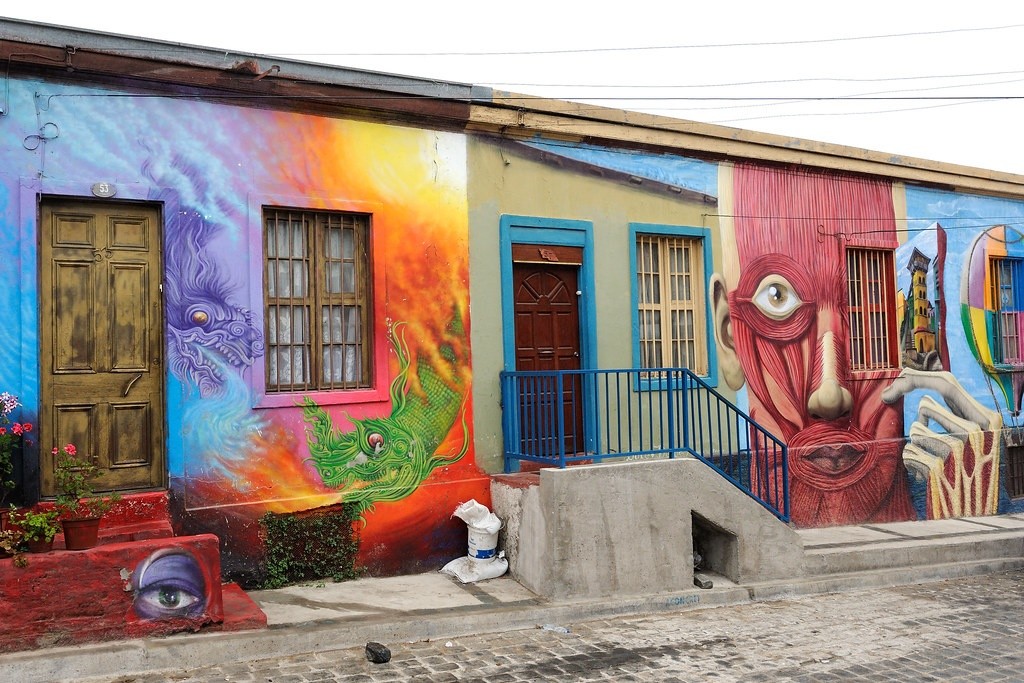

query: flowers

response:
[53,444,121,520]
[0,504,28,568]
[0,392,32,500]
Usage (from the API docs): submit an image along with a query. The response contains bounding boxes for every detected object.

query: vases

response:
[63,521,105,549]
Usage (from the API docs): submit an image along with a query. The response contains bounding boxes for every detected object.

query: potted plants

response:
[23,511,59,553]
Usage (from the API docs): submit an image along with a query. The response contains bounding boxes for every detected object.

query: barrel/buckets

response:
[467,521,505,564]
